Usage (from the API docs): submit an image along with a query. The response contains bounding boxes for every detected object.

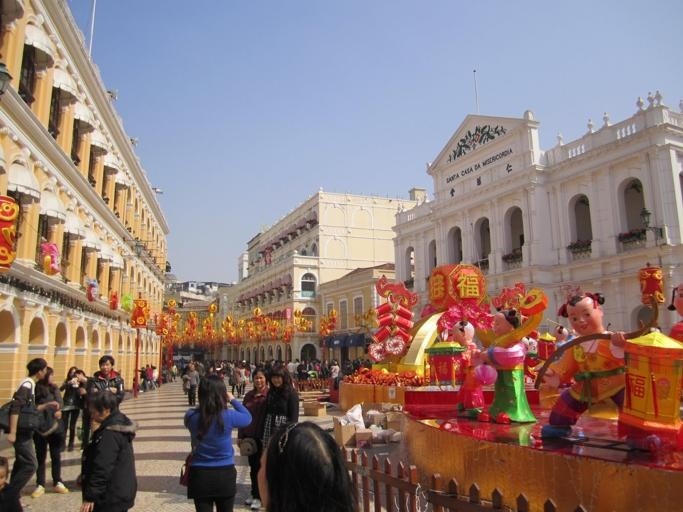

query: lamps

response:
[637,206,663,239]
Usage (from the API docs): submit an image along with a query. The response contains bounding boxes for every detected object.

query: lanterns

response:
[86,279,337,400]
[0,195,19,271]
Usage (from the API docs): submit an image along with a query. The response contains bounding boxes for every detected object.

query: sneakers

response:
[30,485,46,497]
[53,481,69,494]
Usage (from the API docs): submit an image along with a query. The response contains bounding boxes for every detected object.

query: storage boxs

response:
[302,399,402,449]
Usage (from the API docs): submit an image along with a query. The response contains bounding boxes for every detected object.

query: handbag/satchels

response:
[1,400,66,437]
[245,496,262,511]
[239,438,258,457]
[180,454,193,486]
